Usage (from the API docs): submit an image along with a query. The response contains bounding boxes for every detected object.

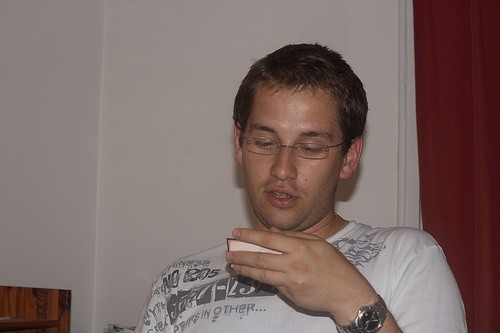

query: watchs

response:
[336,293,388,333]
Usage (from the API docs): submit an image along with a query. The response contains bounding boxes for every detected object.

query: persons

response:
[132,42,471,333]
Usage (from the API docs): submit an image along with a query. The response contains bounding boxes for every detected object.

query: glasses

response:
[238,126,346,159]
